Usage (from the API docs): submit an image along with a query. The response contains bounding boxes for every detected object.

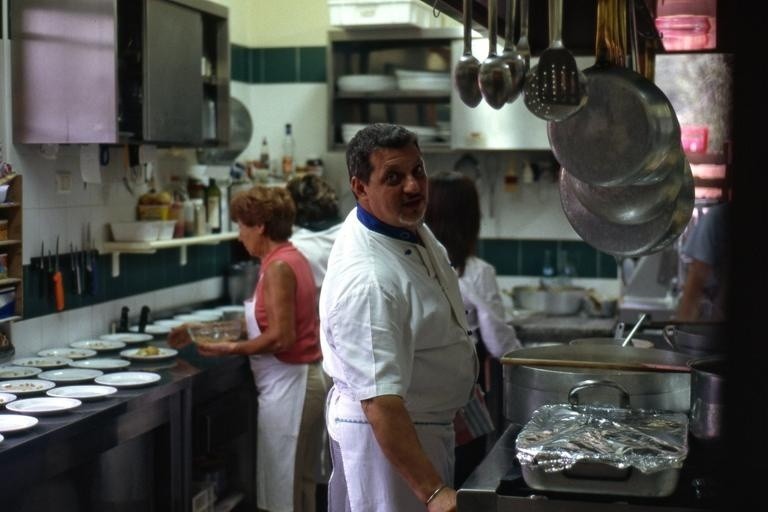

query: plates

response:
[336,66,451,91]
[342,117,450,146]
[131,304,244,333]
[0,332,180,443]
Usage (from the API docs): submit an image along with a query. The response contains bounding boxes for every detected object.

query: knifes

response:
[37,218,101,314]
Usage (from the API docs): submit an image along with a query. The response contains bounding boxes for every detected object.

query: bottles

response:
[172,192,202,237]
[260,121,294,175]
[204,176,223,233]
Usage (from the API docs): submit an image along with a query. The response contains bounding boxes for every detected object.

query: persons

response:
[679,199,732,322]
[318,120,482,511]
[196,187,325,511]
[283,174,343,287]
[422,172,528,369]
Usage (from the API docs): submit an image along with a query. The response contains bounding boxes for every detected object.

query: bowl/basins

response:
[185,319,241,343]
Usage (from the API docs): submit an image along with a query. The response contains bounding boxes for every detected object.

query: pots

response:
[548,0,695,258]
[503,283,618,317]
[686,354,721,443]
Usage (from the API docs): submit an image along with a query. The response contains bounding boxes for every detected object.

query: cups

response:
[307,158,324,178]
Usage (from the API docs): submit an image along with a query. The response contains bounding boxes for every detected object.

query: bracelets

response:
[424,484,447,506]
